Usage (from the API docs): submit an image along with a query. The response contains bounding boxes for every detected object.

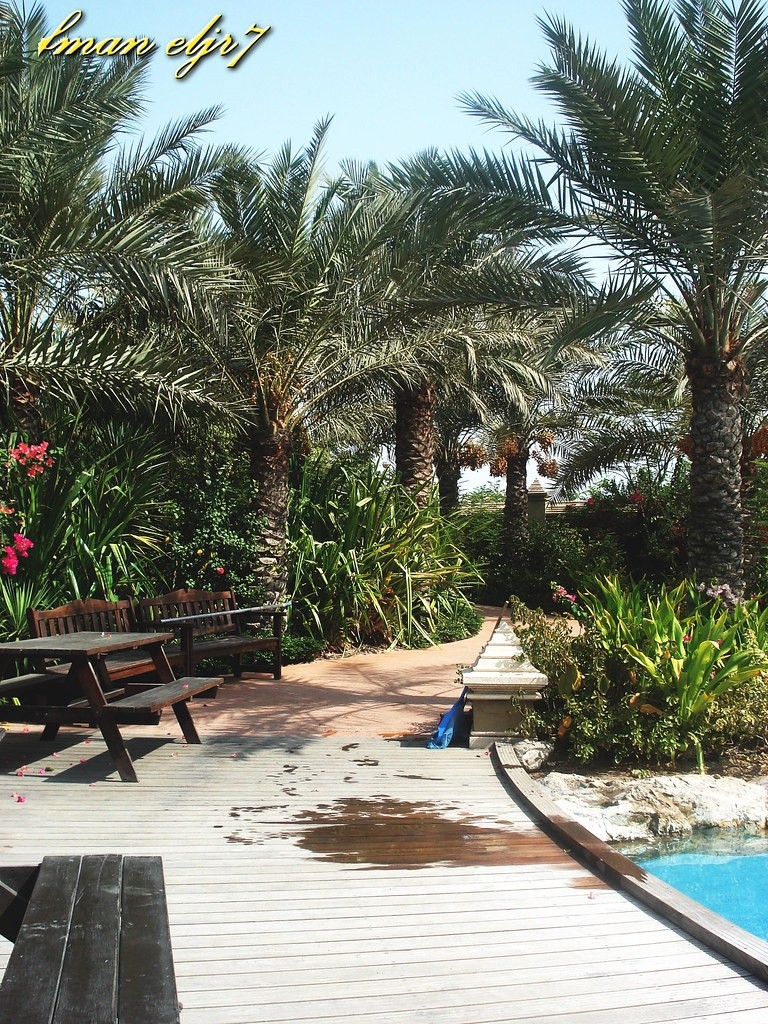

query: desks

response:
[0,630,201,783]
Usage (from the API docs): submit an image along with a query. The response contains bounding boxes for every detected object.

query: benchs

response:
[103,676,223,718]
[0,853,182,1024]
[0,670,65,694]
[134,588,287,702]
[25,593,191,729]
